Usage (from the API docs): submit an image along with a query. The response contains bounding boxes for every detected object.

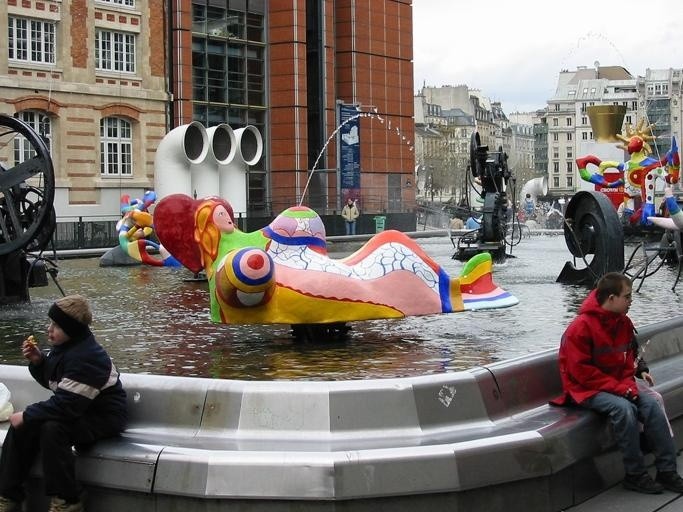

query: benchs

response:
[0,316,682,512]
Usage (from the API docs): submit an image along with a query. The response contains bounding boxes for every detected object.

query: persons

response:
[338,197,360,236]
[0,295,129,511]
[449,214,463,229]
[521,193,535,214]
[466,212,480,230]
[544,271,683,496]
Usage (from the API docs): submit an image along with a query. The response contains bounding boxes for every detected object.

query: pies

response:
[27,336,37,348]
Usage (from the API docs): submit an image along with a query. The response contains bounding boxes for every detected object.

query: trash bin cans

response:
[373,216,386,234]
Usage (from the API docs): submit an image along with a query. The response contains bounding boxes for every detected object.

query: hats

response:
[46,294,94,337]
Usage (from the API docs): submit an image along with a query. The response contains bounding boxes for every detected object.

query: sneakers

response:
[48,496,83,512]
[623,470,664,494]
[655,469,683,493]
[0,497,22,512]
